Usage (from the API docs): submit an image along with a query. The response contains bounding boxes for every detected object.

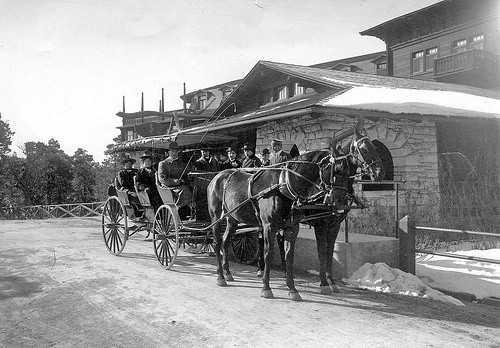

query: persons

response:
[108,180,119,212]
[118,155,144,217]
[117,182,121,190]
[135,154,166,220]
[158,142,196,220]
[151,156,161,173]
[260,148,270,166]
[219,145,242,170]
[269,139,289,168]
[195,143,220,178]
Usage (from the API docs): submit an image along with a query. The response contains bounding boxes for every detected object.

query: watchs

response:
[241,143,263,168]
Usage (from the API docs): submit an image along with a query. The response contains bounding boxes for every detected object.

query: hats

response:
[201,143,213,150]
[260,148,271,155]
[271,138,282,146]
[120,155,136,163]
[241,143,256,150]
[169,141,181,150]
[139,152,152,158]
[226,146,238,152]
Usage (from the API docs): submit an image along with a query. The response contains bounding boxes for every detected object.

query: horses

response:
[255,125,385,294]
[207,142,350,301]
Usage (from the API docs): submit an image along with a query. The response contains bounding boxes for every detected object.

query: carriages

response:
[98,128,385,302]
